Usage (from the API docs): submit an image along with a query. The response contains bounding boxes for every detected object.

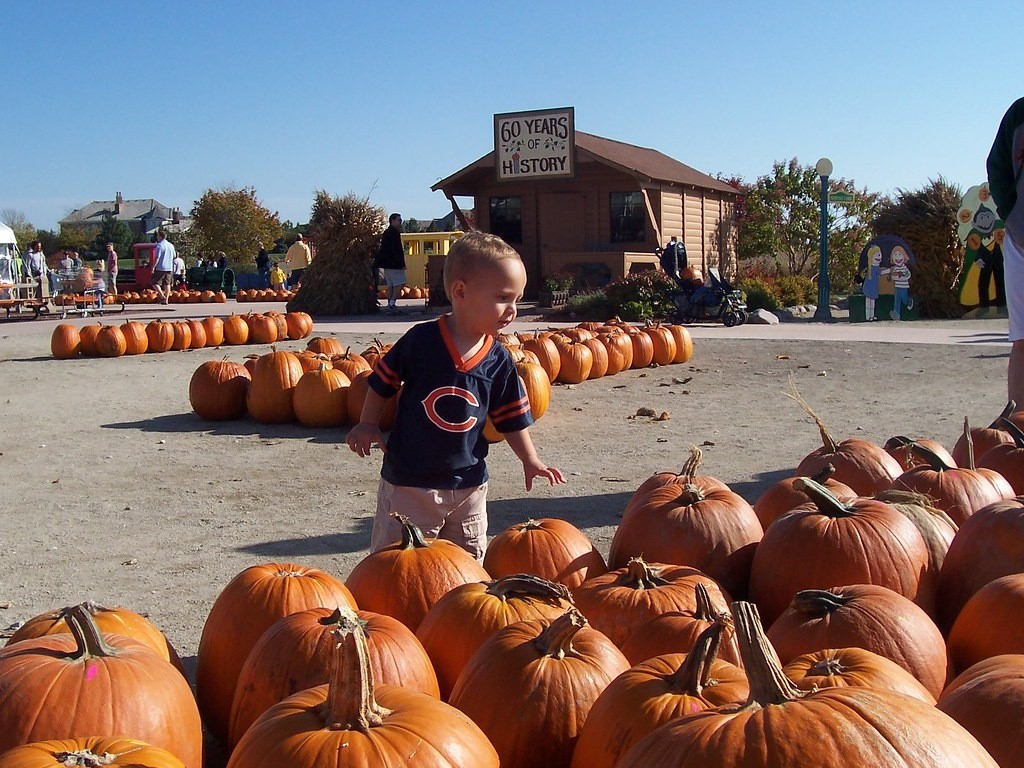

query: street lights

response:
[814,157,834,320]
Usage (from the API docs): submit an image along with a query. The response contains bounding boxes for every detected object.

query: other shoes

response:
[386,305,401,316]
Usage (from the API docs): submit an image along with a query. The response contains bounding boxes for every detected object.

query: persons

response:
[345,230,567,565]
[254,242,270,290]
[107,242,117,295]
[73,259,103,309]
[218,251,229,269]
[58,251,82,271]
[151,230,176,305]
[284,232,312,285]
[286,272,292,286]
[21,241,54,298]
[986,96,1024,413]
[9,250,20,299]
[378,213,407,312]
[196,252,206,267]
[173,251,186,284]
[206,250,217,268]
[270,263,287,290]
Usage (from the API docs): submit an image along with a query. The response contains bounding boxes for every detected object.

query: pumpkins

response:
[482,316,693,443]
[188,337,401,433]
[377,286,429,299]
[0,395,1024,768]
[50,309,313,359]
[55,286,300,305]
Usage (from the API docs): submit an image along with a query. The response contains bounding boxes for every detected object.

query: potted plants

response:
[537,272,575,308]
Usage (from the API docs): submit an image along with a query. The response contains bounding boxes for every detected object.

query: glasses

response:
[394,218,402,221]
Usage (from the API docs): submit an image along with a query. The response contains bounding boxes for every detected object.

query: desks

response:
[0,284,16,299]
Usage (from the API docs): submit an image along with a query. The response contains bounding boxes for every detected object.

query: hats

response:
[63,251,69,255]
[295,232,302,239]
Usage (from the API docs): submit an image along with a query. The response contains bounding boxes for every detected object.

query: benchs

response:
[0,296,53,321]
